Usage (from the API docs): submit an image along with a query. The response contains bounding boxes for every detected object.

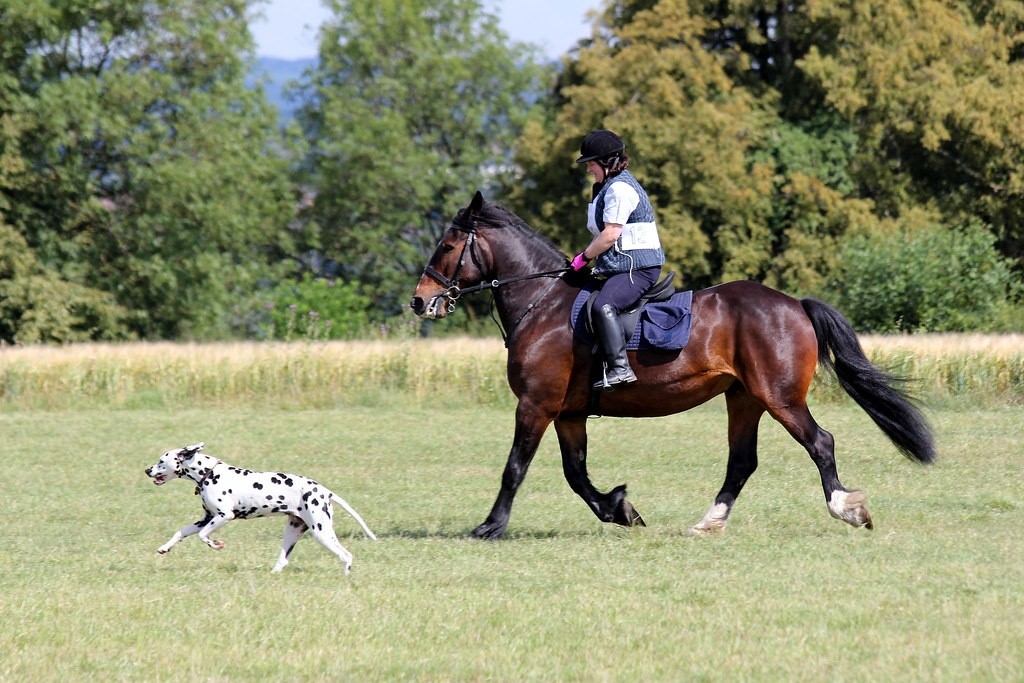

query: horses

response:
[411,190,937,539]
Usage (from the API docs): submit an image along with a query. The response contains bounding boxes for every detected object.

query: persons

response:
[570,130,665,387]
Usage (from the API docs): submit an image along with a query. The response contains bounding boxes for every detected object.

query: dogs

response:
[144,442,377,577]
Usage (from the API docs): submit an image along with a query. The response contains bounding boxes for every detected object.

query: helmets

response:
[576,130,626,163]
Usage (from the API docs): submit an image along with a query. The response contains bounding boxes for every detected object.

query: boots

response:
[593,304,638,390]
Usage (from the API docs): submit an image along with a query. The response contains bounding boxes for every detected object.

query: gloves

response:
[571,250,592,271]
